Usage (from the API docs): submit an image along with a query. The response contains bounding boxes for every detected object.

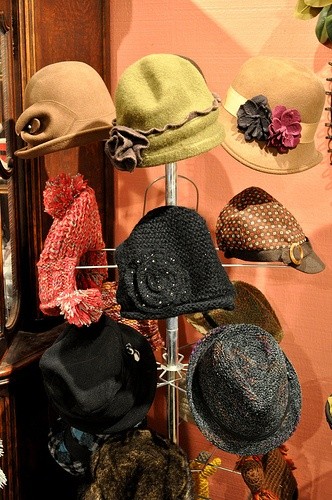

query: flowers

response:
[236,95,273,142]
[269,105,302,153]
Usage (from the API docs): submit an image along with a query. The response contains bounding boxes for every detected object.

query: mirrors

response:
[0,29,19,331]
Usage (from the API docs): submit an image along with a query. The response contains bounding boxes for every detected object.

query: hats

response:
[47,415,141,479]
[34,314,157,436]
[215,57,326,175]
[36,171,108,328]
[233,446,299,500]
[99,281,164,352]
[182,281,284,345]
[78,430,194,500]
[215,187,326,274]
[114,205,235,318]
[186,324,302,457]
[188,450,221,500]
[14,59,117,158]
[103,52,228,172]
